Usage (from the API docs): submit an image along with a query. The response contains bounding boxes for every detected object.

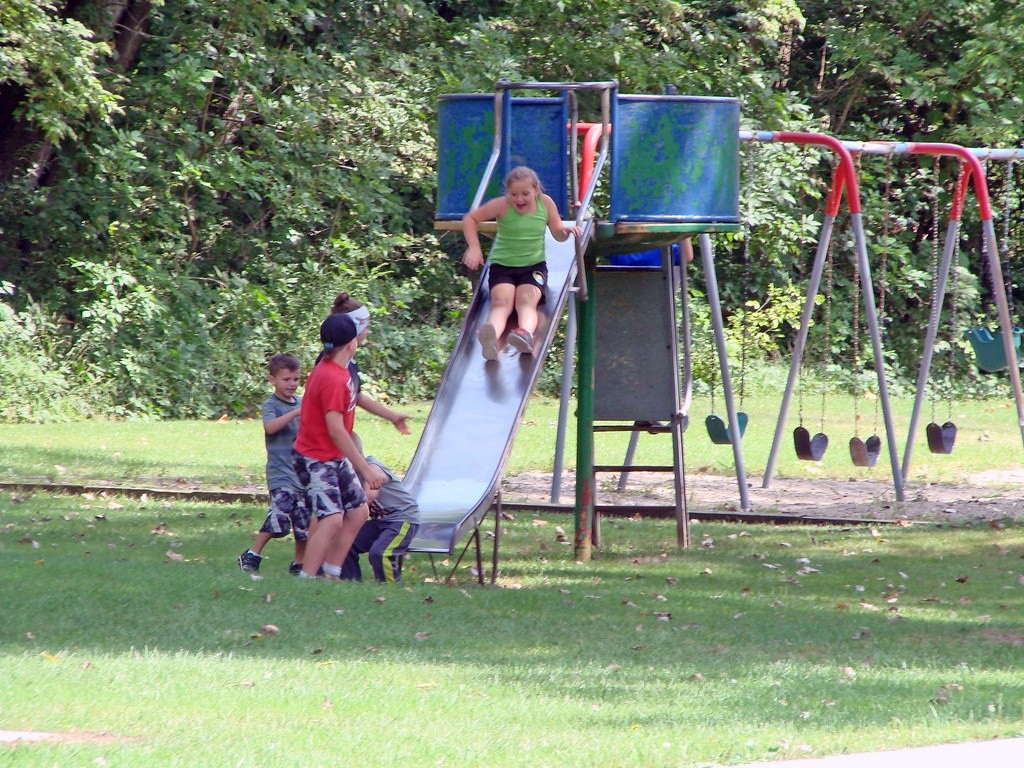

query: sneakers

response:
[289,560,323,576]
[238,549,262,574]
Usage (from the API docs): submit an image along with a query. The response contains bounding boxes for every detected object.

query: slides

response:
[358,219,581,548]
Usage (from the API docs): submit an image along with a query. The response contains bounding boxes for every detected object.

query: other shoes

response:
[478,322,498,362]
[508,328,535,354]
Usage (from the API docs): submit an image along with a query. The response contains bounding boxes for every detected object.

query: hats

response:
[320,312,358,347]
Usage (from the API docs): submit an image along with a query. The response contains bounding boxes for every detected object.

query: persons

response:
[341,431,421,582]
[604,239,693,266]
[462,166,584,361]
[315,293,414,435]
[238,353,312,576]
[301,314,383,579]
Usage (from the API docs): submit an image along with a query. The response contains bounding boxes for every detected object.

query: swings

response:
[925,156,966,454]
[850,153,890,467]
[794,143,829,461]
[705,142,754,446]
[968,162,1024,372]
